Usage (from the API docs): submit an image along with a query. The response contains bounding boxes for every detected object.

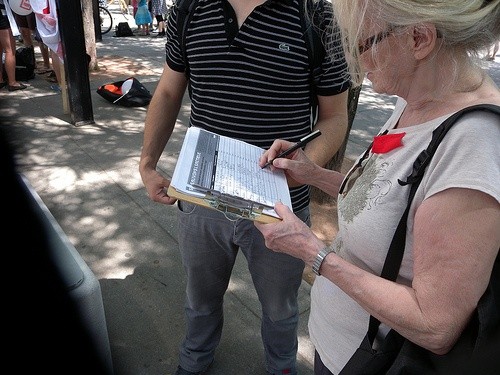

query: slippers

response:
[36,66,52,75]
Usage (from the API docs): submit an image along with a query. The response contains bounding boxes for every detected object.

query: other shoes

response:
[47,76,59,83]
[8,83,27,91]
[0,82,8,88]
[135,27,166,36]
[150,355,216,375]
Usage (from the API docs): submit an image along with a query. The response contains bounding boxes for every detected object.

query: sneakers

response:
[50,83,60,91]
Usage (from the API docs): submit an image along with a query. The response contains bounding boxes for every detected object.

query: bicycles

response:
[99,0,130,22]
[99,6,112,35]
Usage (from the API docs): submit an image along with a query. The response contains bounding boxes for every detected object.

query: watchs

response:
[312,246,337,276]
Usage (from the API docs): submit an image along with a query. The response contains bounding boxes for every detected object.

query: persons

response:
[130,1,167,38]
[254,1,500,375]
[138,1,351,375]
[0,1,67,91]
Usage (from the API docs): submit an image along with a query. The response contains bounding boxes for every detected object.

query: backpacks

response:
[117,22,133,36]
[97,77,152,107]
[338,105,500,375]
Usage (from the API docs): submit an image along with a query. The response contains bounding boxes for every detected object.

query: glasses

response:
[345,25,406,59]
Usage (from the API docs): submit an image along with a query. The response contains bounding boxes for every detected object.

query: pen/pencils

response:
[262,130,322,169]
